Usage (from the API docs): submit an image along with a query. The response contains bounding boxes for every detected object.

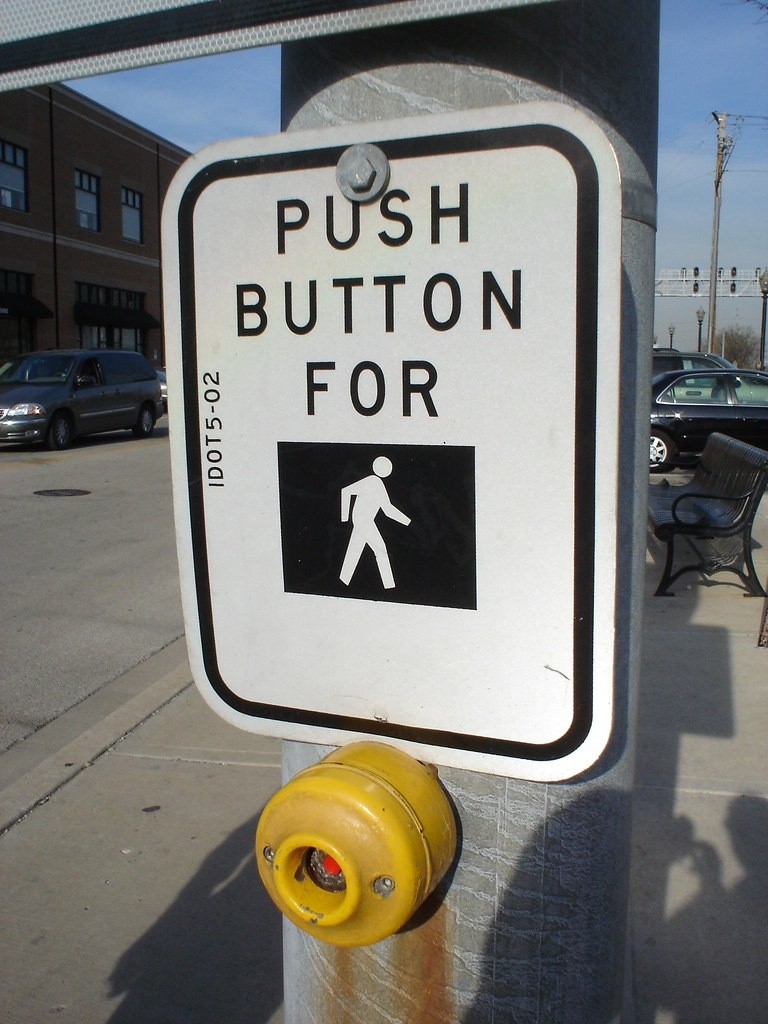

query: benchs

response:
[645,432,768,598]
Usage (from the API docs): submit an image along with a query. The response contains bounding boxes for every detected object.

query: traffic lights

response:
[693,283,698,293]
[730,283,736,292]
[731,267,736,277]
[694,267,699,277]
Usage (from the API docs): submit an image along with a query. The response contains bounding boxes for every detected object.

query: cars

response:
[649,367,768,474]
[156,368,168,413]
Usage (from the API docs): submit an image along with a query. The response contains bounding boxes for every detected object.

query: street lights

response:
[757,265,768,372]
[695,305,706,352]
[668,322,675,373]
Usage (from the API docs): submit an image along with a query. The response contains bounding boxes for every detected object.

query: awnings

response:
[0,294,54,319]
[73,302,162,328]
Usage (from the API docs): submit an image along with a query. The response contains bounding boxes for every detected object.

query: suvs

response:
[652,345,768,402]
[0,349,165,452]
